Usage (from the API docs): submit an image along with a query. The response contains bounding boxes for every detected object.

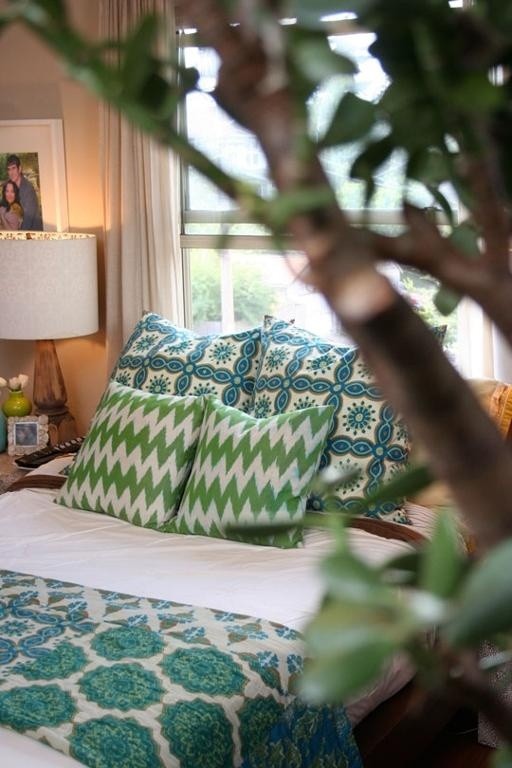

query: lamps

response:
[0,233,99,452]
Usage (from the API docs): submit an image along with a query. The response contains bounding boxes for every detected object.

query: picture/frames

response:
[0,117,71,233]
[8,414,48,454]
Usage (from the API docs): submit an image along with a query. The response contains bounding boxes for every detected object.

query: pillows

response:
[411,375,512,512]
[54,380,206,533]
[164,394,337,551]
[251,315,446,525]
[108,311,294,412]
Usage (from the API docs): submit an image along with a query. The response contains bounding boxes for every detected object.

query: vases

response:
[3,391,32,412]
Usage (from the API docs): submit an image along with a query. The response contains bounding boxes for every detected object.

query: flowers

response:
[1,372,30,395]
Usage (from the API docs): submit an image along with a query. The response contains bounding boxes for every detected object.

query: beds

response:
[1,312,512,764]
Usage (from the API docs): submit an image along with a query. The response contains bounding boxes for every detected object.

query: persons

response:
[21,424,36,445]
[1,180,24,232]
[4,154,42,231]
[15,427,30,445]
